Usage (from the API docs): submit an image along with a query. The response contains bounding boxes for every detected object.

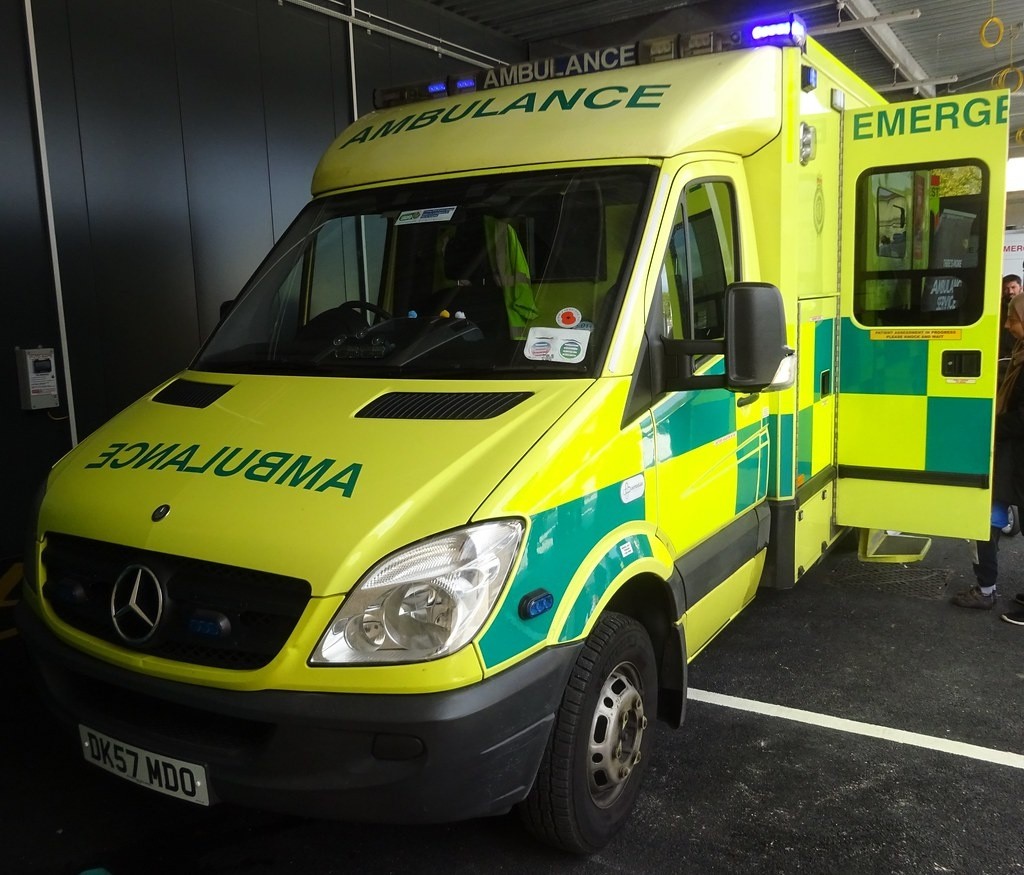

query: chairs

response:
[406,213,540,340]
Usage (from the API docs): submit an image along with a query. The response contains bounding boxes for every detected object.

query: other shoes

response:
[1002,611,1024,625]
[1014,593,1024,607]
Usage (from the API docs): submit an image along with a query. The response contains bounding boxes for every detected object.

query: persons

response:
[949,274,1024,625]
[879,231,906,258]
[435,178,539,368]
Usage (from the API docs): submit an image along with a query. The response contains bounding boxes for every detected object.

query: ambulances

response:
[22,11,1014,859]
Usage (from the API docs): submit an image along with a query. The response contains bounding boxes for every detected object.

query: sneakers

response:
[954,586,997,609]
